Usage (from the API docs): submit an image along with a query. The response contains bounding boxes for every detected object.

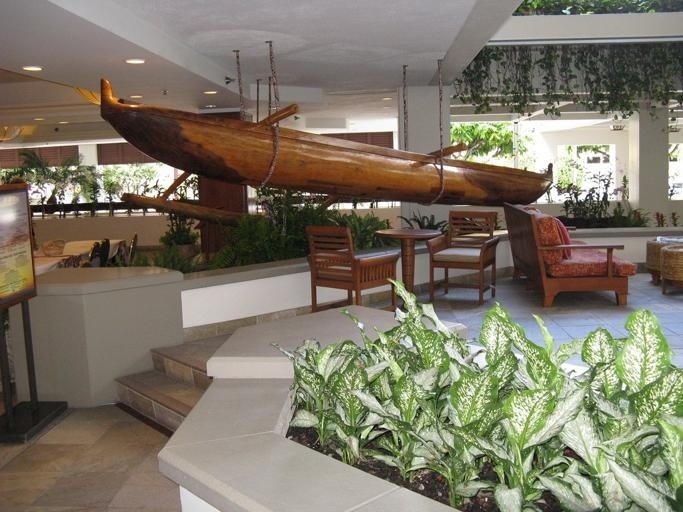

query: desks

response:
[375,229,442,312]
[646,239,683,285]
[660,245,683,294]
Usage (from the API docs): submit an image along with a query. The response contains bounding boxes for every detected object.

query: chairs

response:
[426,210,499,305]
[304,226,399,313]
[42,233,137,267]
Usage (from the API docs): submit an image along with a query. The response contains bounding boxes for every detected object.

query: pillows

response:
[517,204,571,265]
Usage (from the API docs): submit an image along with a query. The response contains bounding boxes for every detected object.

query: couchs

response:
[504,202,638,308]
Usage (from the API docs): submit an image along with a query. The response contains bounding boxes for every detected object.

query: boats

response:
[98,76,552,229]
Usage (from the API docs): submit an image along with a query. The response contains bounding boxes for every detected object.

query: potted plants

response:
[143,176,200,256]
